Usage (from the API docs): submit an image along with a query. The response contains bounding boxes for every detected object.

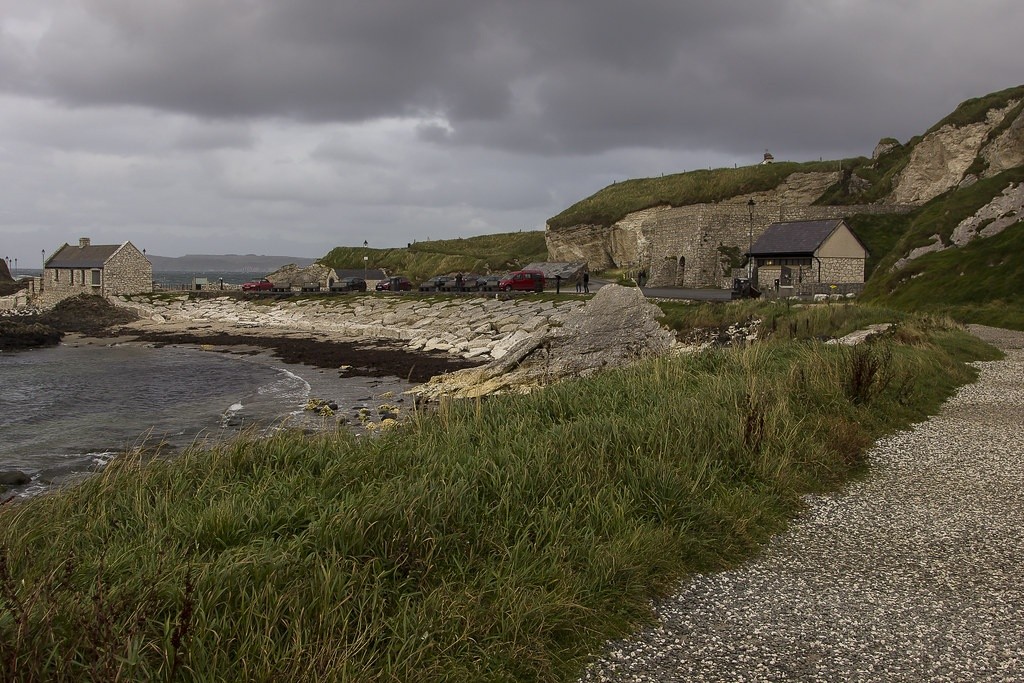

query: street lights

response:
[41,249,45,277]
[5,256,9,266]
[362,240,369,278]
[748,197,755,280]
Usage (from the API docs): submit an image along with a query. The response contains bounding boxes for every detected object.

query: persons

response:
[575,272,582,292]
[455,271,463,291]
[584,270,589,294]
[638,270,646,286]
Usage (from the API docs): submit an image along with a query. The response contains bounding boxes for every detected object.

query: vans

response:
[498,270,546,292]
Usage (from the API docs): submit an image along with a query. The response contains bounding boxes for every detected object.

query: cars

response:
[419,276,454,291]
[376,277,411,291]
[330,277,366,292]
[240,277,274,292]
